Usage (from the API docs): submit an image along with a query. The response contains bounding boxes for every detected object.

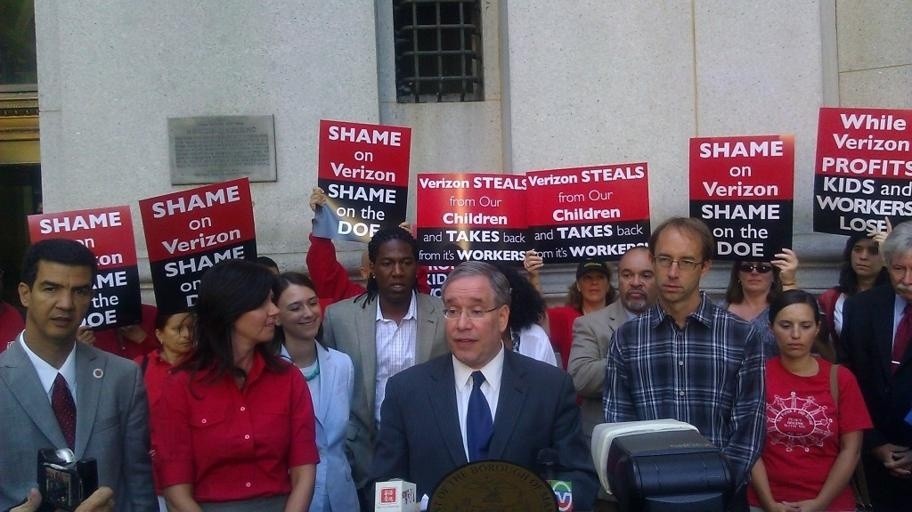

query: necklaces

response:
[302,366,321,381]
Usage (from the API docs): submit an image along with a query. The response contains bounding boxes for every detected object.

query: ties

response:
[48,371,84,457]
[465,370,498,464]
[886,298,912,378]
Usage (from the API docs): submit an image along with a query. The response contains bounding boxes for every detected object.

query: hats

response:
[574,258,613,283]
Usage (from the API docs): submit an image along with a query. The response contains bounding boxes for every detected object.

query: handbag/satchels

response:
[824,361,880,511]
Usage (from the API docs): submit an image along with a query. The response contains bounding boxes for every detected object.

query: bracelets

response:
[783,282,798,287]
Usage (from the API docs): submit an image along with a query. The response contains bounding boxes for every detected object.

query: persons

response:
[601,213,768,511]
[714,247,800,357]
[813,214,891,363]
[523,249,620,409]
[6,483,118,512]
[147,258,323,512]
[131,308,199,510]
[0,237,166,510]
[839,219,912,510]
[78,303,162,360]
[267,270,363,511]
[745,287,874,511]
[565,243,660,448]
[0,267,26,356]
[497,263,559,370]
[305,184,432,319]
[255,255,281,278]
[375,258,601,511]
[318,224,450,511]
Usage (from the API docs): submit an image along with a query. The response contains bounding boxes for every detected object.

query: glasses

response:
[650,254,704,272]
[735,262,774,275]
[440,303,502,322]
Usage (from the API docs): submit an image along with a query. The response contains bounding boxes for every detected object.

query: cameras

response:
[37,447,99,512]
